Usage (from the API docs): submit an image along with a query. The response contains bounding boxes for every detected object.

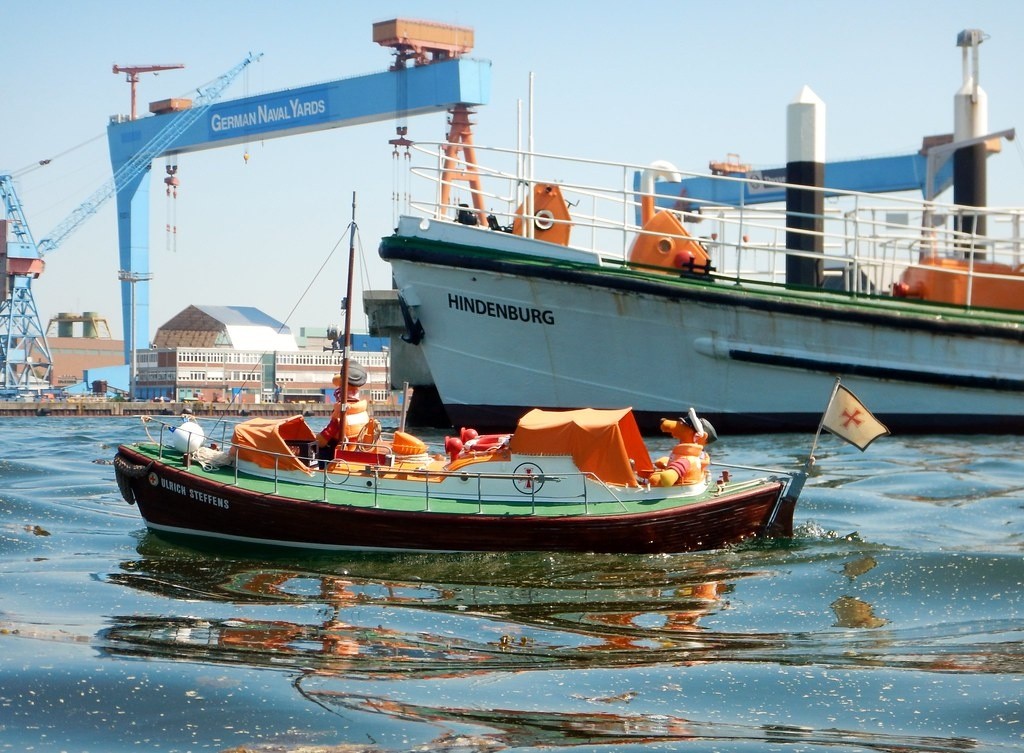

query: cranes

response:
[1,50,267,280]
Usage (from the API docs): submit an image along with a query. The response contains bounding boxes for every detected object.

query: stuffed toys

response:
[313,358,369,463]
[649,411,717,488]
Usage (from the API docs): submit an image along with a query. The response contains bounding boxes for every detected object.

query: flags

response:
[823,386,891,451]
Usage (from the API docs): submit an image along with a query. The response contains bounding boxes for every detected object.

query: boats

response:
[378,24,1024,444]
[114,191,889,559]
